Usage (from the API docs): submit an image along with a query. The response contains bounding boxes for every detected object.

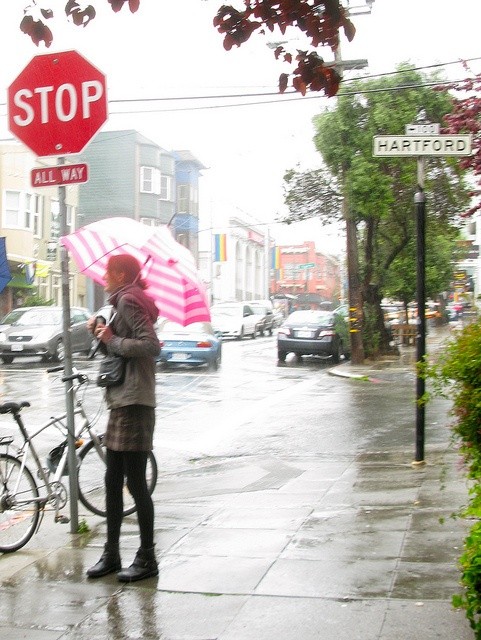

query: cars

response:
[0,307,91,364]
[207,302,257,341]
[153,322,221,373]
[255,301,275,336]
[277,310,348,364]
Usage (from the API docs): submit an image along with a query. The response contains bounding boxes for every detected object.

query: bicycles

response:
[2,366,157,553]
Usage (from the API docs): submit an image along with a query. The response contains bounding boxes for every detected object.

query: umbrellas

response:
[56,211,212,361]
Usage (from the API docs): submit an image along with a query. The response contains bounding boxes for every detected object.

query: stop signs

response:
[6,50,107,158]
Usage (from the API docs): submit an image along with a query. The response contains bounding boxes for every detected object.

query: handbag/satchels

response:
[96,355,127,387]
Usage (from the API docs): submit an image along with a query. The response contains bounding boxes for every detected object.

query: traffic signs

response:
[371,123,472,156]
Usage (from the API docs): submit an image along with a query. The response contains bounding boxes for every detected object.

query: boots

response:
[86,542,121,577]
[118,544,158,582]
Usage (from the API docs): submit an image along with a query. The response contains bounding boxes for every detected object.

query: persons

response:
[83,253,165,585]
